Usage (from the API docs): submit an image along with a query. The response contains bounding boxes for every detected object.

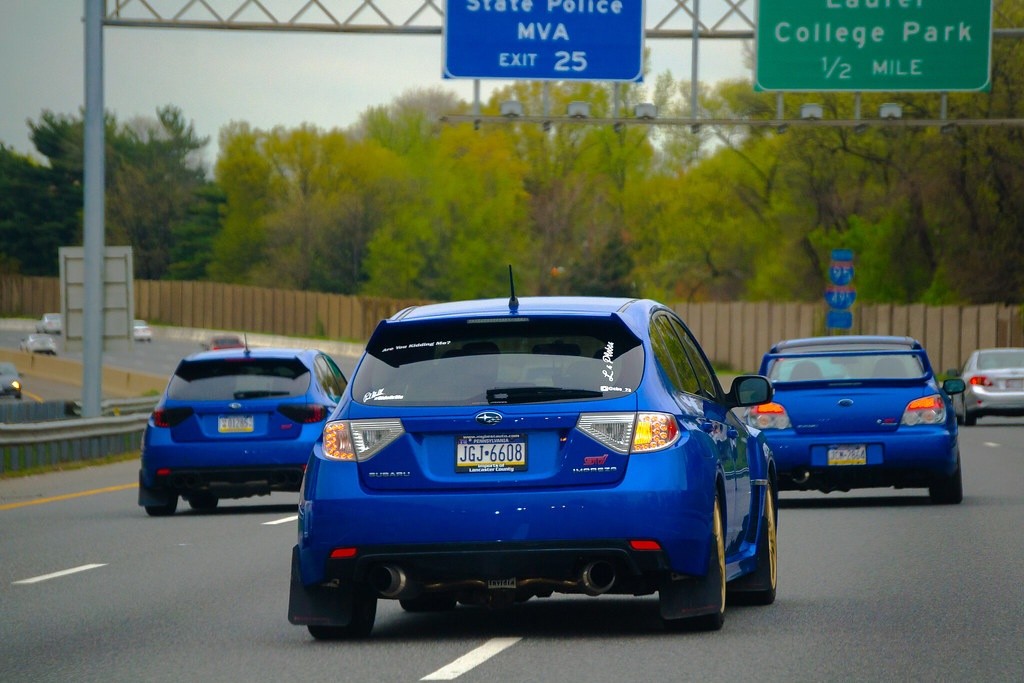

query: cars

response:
[743,336,967,503]
[0,363,24,399]
[35,312,62,335]
[953,346,1024,426]
[201,336,246,351]
[134,320,151,341]
[137,348,349,517]
[287,297,780,642]
[20,334,56,356]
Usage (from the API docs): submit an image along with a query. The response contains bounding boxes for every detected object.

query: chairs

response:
[790,362,823,380]
[429,342,620,403]
[871,358,906,378]
